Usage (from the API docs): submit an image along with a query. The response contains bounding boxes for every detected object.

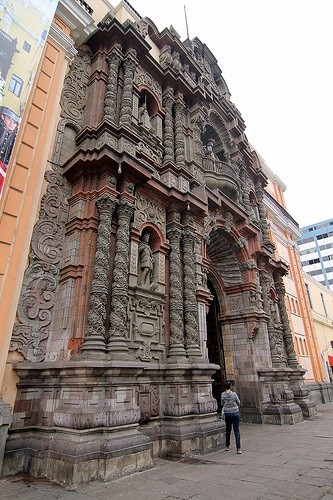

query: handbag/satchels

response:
[221,408,225,420]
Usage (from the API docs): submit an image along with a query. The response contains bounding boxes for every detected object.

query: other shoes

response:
[225,446,230,451]
[237,448,242,454]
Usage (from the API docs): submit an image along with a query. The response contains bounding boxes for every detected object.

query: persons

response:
[202,138,222,172]
[139,102,151,129]
[268,293,275,322]
[218,382,242,455]
[0,106,19,164]
[137,233,156,290]
